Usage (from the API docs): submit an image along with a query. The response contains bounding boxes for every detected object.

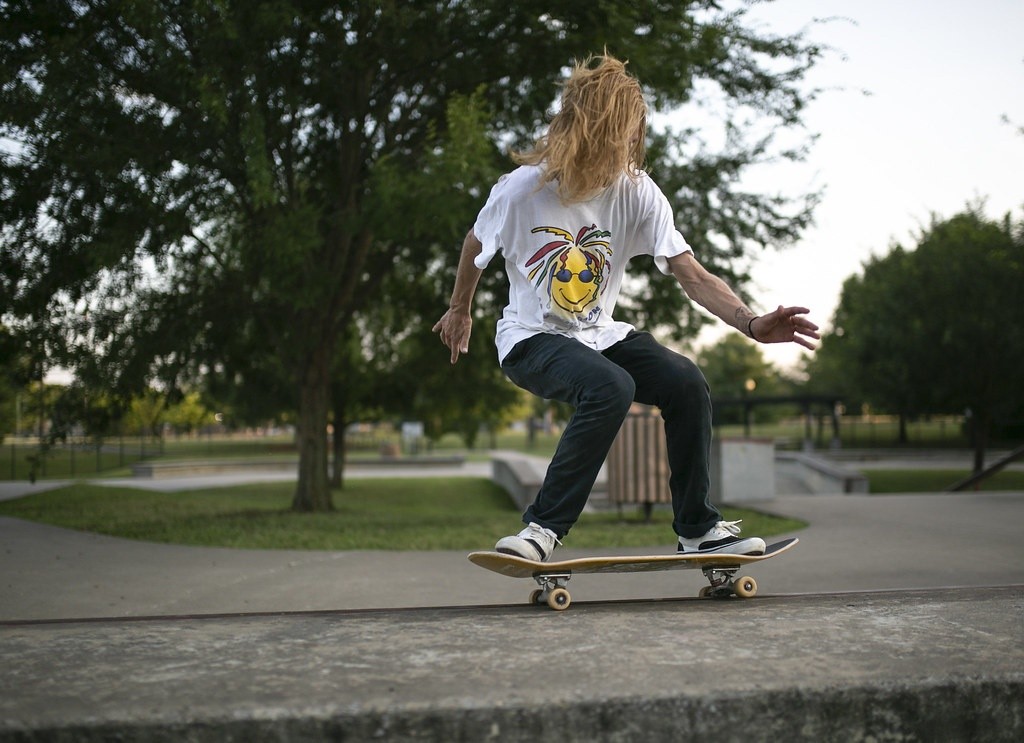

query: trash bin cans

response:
[608,412,671,505]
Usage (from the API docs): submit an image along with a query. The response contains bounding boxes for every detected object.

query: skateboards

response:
[466,536,801,611]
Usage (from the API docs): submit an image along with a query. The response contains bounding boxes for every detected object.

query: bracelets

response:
[750,316,760,343]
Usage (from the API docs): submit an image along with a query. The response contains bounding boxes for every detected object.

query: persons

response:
[431,44,821,563]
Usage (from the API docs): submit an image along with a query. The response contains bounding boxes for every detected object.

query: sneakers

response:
[496,522,563,562]
[677,519,766,556]
